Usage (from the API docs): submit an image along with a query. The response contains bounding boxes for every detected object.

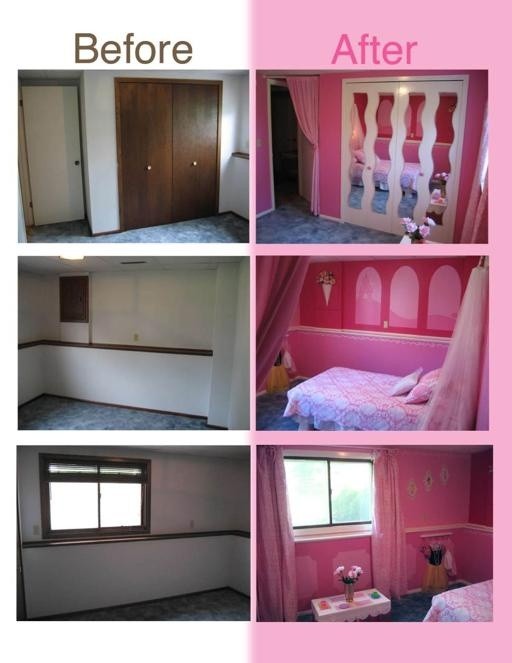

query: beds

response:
[287,366,426,431]
[349,156,422,200]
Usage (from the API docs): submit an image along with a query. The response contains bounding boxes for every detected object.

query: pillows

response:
[354,149,380,169]
[392,366,442,404]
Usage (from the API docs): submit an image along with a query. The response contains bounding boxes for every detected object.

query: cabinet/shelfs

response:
[59,272,90,323]
[340,75,471,243]
[115,77,223,234]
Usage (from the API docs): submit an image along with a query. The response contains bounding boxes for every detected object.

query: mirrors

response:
[347,92,455,228]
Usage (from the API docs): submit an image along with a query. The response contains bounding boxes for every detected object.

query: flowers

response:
[314,271,336,284]
[433,169,449,185]
[332,565,363,584]
[400,216,436,240]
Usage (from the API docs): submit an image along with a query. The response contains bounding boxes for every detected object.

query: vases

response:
[440,185,446,197]
[410,239,426,243]
[345,582,355,602]
